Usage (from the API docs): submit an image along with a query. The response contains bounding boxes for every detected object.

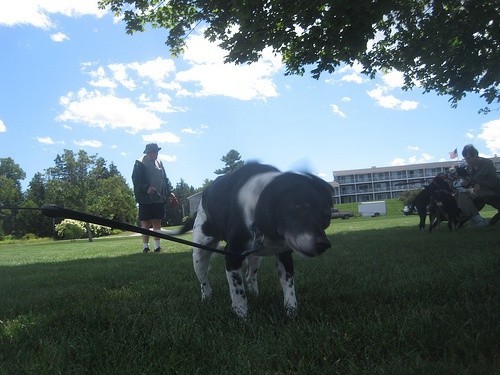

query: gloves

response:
[149,187,160,201]
[168,193,180,208]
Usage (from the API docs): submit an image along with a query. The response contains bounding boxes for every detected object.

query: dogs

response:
[402,166,487,232]
[154,160,335,320]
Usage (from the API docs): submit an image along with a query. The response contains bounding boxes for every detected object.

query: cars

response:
[331,208,354,220]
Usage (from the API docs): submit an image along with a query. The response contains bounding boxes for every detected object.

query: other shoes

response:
[143,247,150,253]
[154,246,162,252]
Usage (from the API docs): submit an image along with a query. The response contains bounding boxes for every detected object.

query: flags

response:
[451,149,457,158]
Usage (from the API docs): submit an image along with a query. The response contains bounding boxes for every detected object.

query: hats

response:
[143,143,162,153]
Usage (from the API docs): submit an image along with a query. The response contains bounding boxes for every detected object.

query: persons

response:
[132,144,179,252]
[456,145,500,225]
